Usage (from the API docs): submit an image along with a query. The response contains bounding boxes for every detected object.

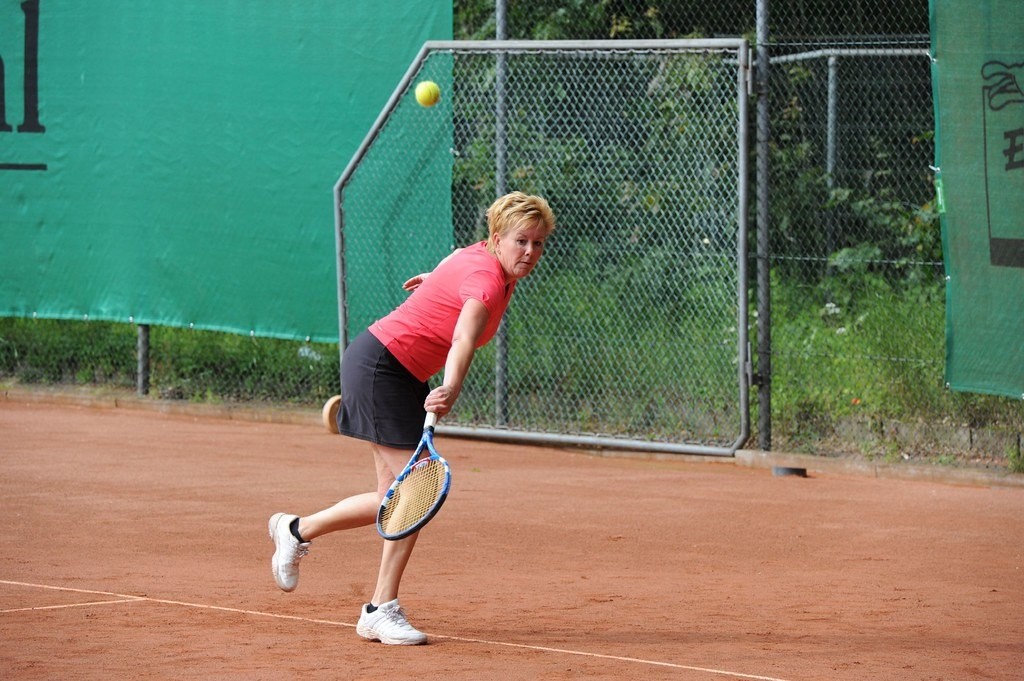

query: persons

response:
[268,191,554,648]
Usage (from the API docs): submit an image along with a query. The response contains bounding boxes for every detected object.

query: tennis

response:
[414,81,439,107]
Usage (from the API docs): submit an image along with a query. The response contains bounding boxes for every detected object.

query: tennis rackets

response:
[375,408,450,541]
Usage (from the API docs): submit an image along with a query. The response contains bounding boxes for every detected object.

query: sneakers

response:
[269,512,311,591]
[356,598,429,644]
